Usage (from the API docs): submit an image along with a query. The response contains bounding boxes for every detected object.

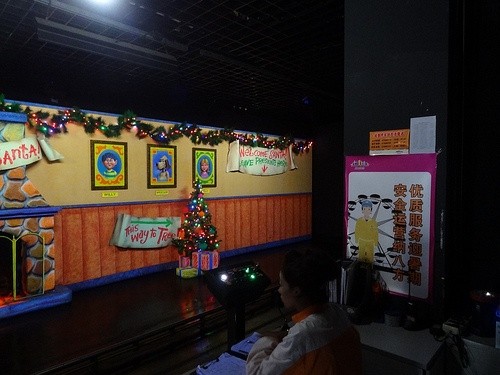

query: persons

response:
[245,266,363,375]
